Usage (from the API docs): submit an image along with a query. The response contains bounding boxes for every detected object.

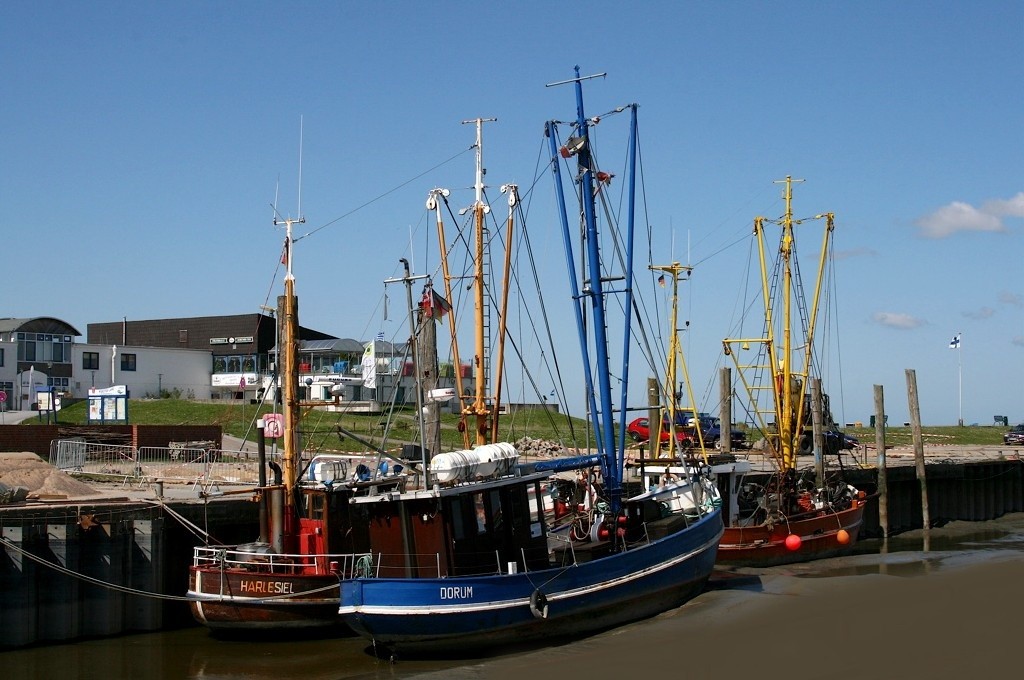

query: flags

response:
[423,287,453,325]
[948,335,960,348]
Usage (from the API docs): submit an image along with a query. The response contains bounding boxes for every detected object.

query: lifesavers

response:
[529,588,549,620]
[657,473,680,488]
[265,417,281,433]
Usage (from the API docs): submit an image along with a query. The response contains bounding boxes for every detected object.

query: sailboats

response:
[185,116,870,634]
[335,63,729,656]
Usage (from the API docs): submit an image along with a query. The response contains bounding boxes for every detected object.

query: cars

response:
[1003,424,1024,445]
[627,410,748,451]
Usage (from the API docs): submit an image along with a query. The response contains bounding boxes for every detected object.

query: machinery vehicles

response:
[752,358,861,456]
